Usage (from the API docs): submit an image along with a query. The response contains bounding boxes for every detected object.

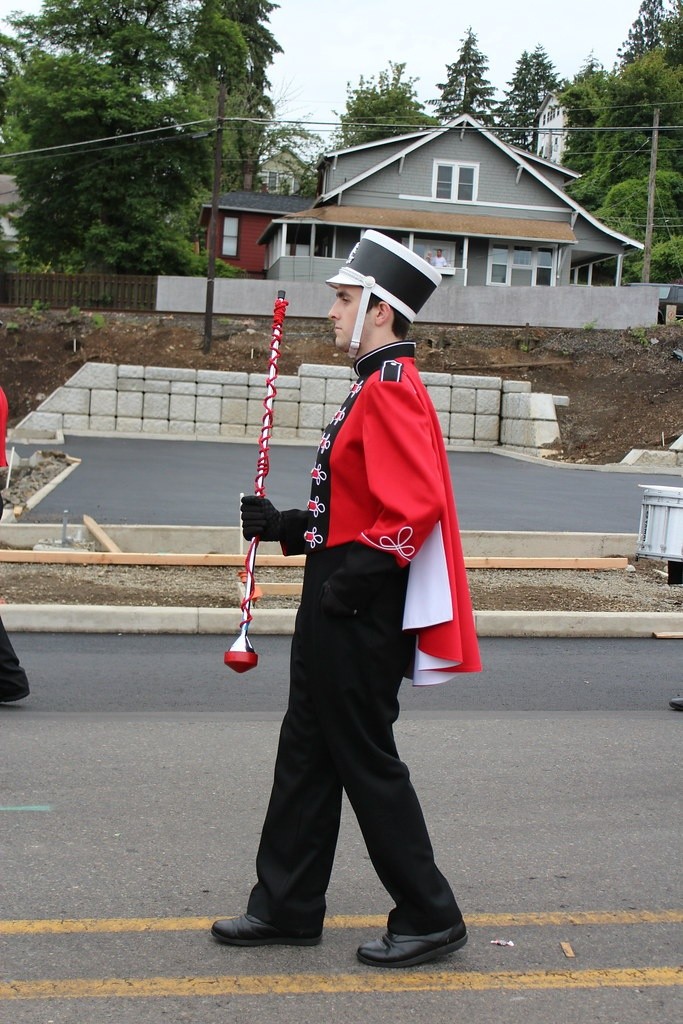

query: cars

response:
[622,281,683,326]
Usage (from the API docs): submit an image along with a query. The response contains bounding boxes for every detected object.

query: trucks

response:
[636,482,683,565]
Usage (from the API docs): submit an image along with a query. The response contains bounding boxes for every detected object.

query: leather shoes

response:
[356,917,469,968]
[212,912,323,946]
[668,697,682,712]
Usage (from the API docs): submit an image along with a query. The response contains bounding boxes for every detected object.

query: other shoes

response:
[0,689,30,702]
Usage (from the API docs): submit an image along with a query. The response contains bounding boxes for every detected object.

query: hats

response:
[324,229,443,326]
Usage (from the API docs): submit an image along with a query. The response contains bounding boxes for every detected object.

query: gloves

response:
[318,580,361,622]
[240,495,281,542]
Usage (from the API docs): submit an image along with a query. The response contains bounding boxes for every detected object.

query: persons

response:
[425,249,447,267]
[212,230,483,968]
[1,389,31,702]
